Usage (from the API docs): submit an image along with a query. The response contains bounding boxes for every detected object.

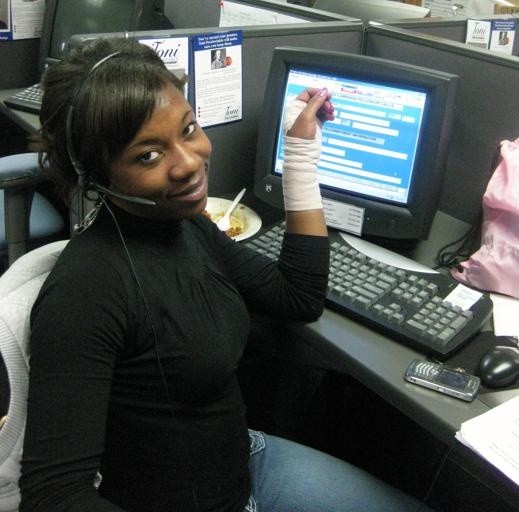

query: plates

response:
[204,197,263,242]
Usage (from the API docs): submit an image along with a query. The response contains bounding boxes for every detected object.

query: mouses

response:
[478,346,519,388]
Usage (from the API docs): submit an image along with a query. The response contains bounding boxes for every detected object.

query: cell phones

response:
[404,359,480,402]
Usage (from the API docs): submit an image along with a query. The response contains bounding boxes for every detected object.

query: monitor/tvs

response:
[252,46,459,249]
[36,0,174,78]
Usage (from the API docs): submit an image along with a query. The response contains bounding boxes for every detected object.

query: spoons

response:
[217,187,248,231]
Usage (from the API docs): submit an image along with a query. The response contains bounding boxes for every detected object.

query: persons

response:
[212,50,226,70]
[16,38,417,510]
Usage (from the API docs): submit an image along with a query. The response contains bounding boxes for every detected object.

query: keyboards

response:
[241,219,493,355]
[3,81,44,112]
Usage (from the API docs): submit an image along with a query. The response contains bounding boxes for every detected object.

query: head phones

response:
[64,52,123,192]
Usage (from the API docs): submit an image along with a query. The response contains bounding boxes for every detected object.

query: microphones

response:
[95,183,157,207]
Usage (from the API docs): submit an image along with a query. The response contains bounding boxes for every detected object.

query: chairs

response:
[0,143,64,269]
[0,243,318,512]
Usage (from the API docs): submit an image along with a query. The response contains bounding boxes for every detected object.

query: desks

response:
[0,86,43,142]
[206,188,519,504]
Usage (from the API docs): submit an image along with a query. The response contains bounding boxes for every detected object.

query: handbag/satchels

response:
[435,138,519,301]
